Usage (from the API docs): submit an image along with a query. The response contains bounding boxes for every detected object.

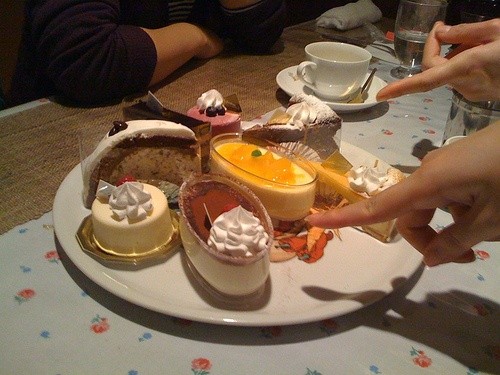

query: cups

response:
[445,10,494,54]
[297,42,372,101]
[443,94,500,141]
[390,0,448,79]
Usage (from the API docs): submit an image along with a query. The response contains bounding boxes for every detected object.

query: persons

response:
[375,18,500,104]
[304,121,500,268]
[10,0,285,109]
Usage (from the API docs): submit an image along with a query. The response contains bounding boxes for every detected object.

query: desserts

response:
[80,89,405,301]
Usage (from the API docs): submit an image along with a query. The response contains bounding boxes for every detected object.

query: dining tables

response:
[0,14,500,375]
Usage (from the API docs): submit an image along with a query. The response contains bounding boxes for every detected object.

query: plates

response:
[75,208,181,265]
[276,64,389,113]
[52,141,424,325]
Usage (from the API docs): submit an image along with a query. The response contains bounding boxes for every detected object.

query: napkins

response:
[317,0,383,31]
